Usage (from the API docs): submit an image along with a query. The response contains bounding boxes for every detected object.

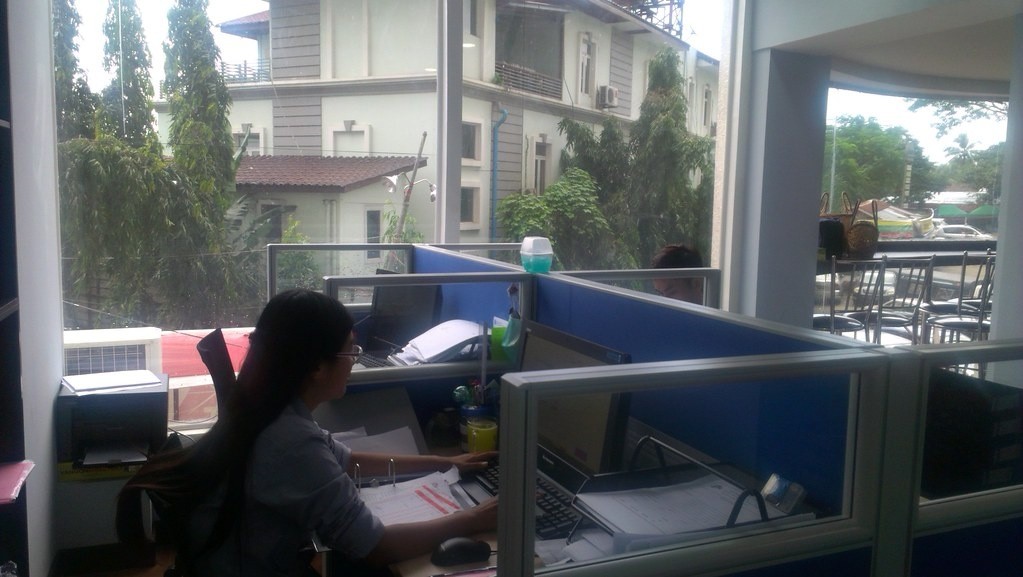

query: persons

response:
[651,245,707,306]
[115,288,500,577]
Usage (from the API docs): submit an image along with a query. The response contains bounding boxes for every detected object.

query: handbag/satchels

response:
[820,217,844,257]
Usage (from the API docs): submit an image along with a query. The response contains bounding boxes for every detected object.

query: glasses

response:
[317,345,363,362]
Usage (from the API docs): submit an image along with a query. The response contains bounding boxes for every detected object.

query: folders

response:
[310,385,476,553]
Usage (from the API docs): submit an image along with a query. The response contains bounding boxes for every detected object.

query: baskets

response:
[821,193,853,247]
[847,198,880,255]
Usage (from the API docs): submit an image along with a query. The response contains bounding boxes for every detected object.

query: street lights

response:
[380,130,439,273]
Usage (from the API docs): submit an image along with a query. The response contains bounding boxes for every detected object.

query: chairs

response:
[816,248,999,377]
[196,329,238,422]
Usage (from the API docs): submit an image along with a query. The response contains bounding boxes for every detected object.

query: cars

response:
[807,287,993,381]
[933,225,993,241]
[815,272,843,304]
[850,270,898,303]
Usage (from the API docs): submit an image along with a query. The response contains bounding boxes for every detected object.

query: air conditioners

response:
[57,324,164,393]
[598,84,620,108]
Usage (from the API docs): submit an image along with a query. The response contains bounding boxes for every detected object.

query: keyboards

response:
[357,353,396,368]
[458,454,602,538]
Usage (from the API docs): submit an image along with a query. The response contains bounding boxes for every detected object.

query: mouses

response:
[431,537,492,566]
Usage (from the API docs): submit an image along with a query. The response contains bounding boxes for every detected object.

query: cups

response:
[467,416,499,457]
[458,404,482,452]
[519,236,553,273]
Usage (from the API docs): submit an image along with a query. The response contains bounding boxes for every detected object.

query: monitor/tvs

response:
[516,320,631,484]
[366,270,443,352]
